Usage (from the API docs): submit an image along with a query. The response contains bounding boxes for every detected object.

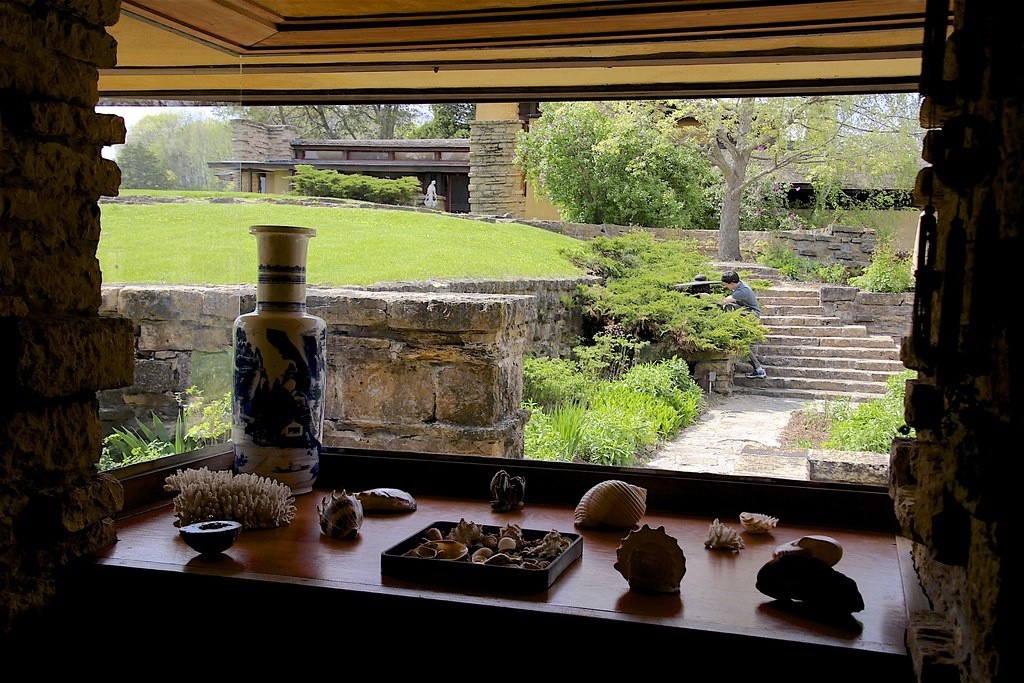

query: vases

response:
[230,226,327,497]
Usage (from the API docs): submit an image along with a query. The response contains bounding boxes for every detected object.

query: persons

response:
[699,270,767,379]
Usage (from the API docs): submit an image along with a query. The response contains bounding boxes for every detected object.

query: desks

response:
[85,477,910,660]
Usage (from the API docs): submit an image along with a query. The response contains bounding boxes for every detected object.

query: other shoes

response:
[744,368,767,379]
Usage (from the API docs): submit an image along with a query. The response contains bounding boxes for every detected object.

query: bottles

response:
[232,225,328,496]
